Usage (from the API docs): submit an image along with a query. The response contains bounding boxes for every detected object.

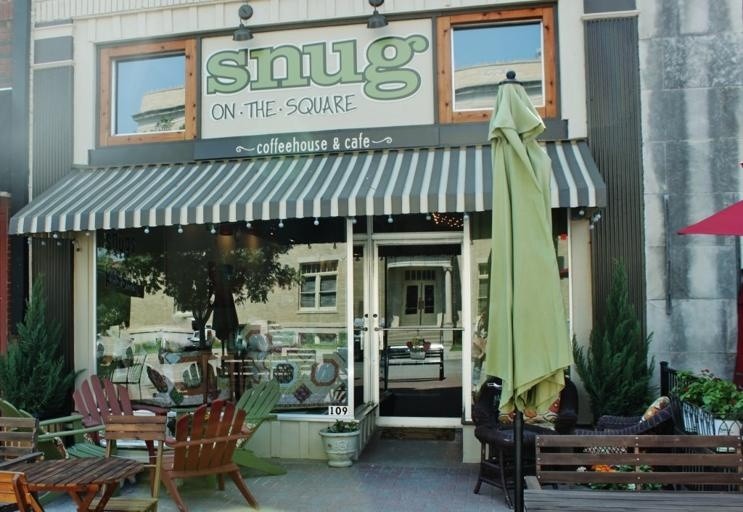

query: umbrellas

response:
[678,201,743,239]
[486,69,577,511]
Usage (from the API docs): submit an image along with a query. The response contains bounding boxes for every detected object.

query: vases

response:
[682,402,742,453]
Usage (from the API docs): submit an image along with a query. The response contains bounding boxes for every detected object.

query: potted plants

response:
[0,272,86,460]
[318,419,360,468]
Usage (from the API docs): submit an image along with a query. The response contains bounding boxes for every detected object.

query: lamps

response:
[366,0,388,28]
[232,5,254,41]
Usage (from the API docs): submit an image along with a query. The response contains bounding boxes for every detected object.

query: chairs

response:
[0,374,266,512]
[472,373,676,510]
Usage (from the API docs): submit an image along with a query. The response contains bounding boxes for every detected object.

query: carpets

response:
[379,427,457,441]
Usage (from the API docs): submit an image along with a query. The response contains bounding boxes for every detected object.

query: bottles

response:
[165,411,176,439]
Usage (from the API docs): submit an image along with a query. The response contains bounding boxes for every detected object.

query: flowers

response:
[672,368,743,420]
